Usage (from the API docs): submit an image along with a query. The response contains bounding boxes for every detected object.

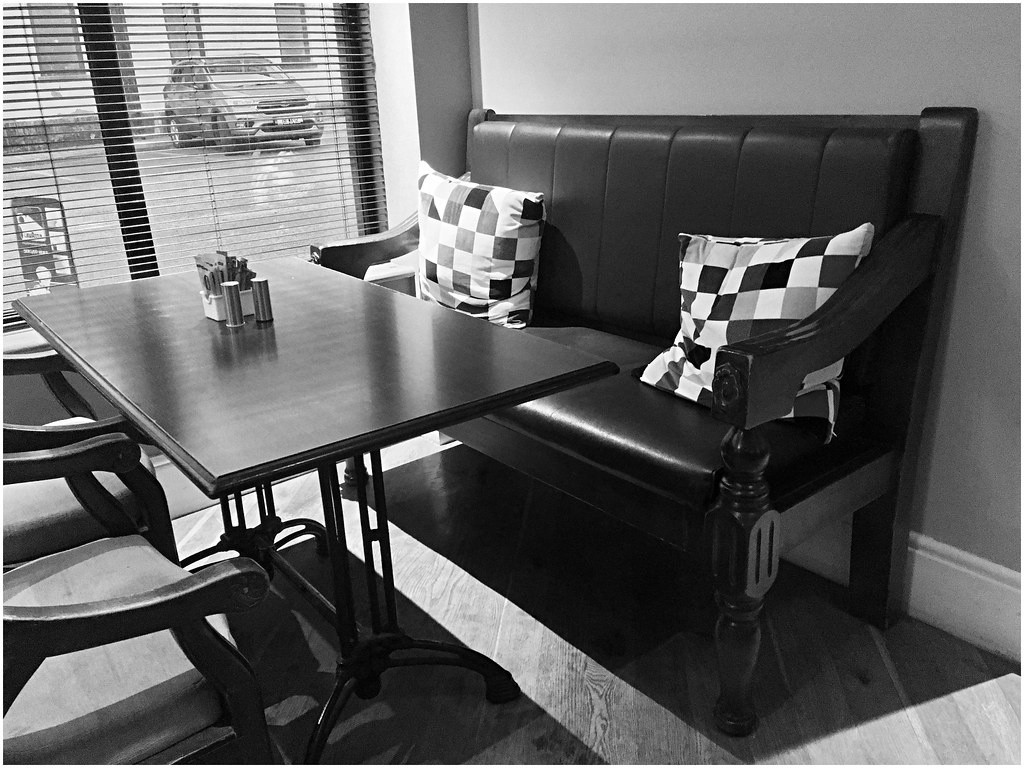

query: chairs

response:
[3,348,270,765]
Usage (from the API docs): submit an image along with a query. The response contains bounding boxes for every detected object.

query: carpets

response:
[341,445,1021,765]
[191,535,609,765]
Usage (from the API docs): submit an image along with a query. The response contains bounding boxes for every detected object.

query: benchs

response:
[306,108,977,738]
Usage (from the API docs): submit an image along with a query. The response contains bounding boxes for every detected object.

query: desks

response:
[4,252,619,765]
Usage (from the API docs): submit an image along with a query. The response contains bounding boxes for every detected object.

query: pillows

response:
[415,161,547,330]
[639,222,874,449]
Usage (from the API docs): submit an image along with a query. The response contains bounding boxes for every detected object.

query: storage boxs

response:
[199,287,255,322]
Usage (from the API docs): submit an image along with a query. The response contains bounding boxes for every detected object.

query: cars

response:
[162,53,325,156]
